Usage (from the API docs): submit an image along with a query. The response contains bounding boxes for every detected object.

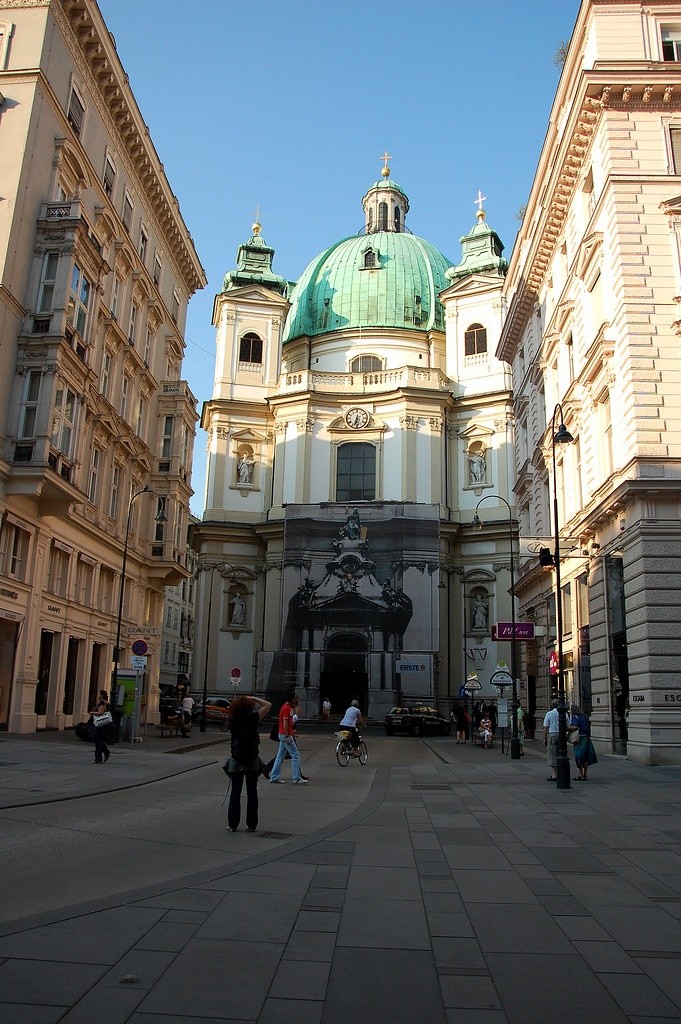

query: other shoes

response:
[301,777,308,780]
[546,776,556,782]
[104,752,111,762]
[228,825,237,832]
[455,740,466,744]
[92,760,102,764]
[263,772,270,779]
[247,825,258,832]
[519,752,524,756]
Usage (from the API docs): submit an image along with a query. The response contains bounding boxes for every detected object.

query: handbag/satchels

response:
[565,728,579,744]
[93,700,113,727]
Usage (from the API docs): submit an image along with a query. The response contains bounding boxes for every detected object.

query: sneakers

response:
[292,777,308,783]
[270,778,284,783]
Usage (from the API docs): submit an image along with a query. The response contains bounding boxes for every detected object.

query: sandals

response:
[573,775,588,780]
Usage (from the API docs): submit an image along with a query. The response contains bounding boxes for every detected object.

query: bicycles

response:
[335,725,368,767]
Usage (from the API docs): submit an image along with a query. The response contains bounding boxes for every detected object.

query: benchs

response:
[472,733,497,749]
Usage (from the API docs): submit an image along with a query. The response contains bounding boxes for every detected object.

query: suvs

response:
[193,697,230,725]
[385,703,451,737]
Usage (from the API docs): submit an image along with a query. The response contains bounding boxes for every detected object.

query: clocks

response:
[344,406,369,430]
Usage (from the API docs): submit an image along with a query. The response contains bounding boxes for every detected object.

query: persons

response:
[339,700,366,756]
[517,701,524,756]
[466,452,485,482]
[230,593,245,623]
[323,698,332,720]
[451,702,466,744]
[473,594,488,629]
[90,690,110,764]
[571,704,598,781]
[263,692,310,784]
[227,696,272,832]
[238,453,255,483]
[474,700,498,749]
[182,694,195,732]
[543,699,570,781]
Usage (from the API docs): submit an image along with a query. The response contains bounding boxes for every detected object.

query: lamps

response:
[591,543,600,549]
[583,550,589,555]
[539,547,591,568]
[620,518,626,531]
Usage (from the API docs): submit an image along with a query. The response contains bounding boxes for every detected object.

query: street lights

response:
[437,566,468,713]
[110,489,168,712]
[552,403,575,788]
[472,495,521,759]
[200,562,237,732]
